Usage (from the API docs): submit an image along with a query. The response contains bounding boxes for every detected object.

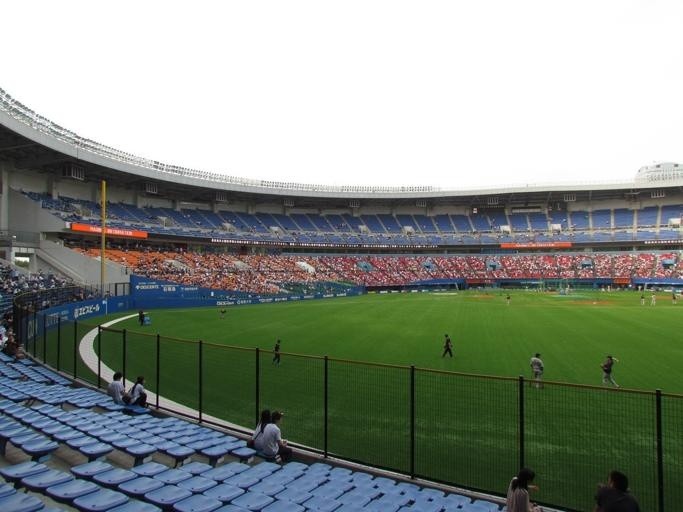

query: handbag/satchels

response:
[122,394,131,404]
[247,437,255,448]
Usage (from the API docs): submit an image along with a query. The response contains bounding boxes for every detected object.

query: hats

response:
[272,411,285,419]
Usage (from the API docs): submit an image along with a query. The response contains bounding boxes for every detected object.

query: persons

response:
[507,295,510,304]
[593,470,641,512]
[530,353,545,389]
[442,334,453,358]
[108,372,126,405]
[221,308,226,319]
[600,355,620,388]
[1,262,110,360]
[506,468,543,512]
[122,376,147,407]
[272,339,281,364]
[640,293,677,307]
[25,190,682,296]
[139,310,145,325]
[262,410,292,462]
[247,410,271,450]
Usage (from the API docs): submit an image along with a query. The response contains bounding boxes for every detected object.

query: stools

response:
[0,286,95,328]
